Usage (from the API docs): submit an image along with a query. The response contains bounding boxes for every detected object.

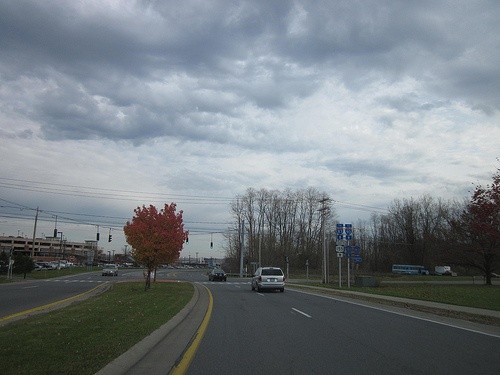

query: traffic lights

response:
[108,234,112,243]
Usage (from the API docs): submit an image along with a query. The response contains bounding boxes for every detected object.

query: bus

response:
[391,263,429,276]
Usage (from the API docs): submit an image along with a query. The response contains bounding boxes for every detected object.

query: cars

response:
[208,268,227,282]
[32,258,86,271]
[100,263,119,277]
[250,266,286,294]
